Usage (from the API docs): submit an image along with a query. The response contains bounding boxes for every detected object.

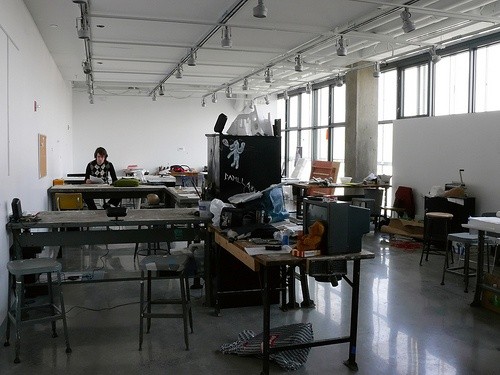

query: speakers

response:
[214,113,228,134]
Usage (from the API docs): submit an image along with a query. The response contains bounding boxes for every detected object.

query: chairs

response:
[54,192,89,231]
[379,186,412,222]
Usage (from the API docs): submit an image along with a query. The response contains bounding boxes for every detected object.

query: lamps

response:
[71,0,441,107]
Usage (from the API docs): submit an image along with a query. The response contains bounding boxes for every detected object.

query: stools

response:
[133,202,194,350]
[351,198,376,212]
[2,259,73,363]
[420,211,500,293]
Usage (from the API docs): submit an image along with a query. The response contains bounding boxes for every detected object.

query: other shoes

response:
[103,204,111,209]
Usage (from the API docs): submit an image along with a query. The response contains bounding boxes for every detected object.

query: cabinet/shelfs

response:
[425,196,476,250]
[205,133,282,310]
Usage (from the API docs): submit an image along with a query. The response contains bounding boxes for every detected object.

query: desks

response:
[165,187,202,208]
[48,184,167,209]
[469,217,500,308]
[290,183,391,221]
[170,172,199,186]
[144,175,176,208]
[206,222,375,375]
[8,210,215,290]
[63,176,141,185]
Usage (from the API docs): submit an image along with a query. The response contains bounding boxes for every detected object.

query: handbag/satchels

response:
[220,322,314,372]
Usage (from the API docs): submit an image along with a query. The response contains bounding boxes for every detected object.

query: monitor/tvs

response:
[303,198,370,256]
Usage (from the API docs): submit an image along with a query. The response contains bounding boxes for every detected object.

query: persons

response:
[82,147,122,210]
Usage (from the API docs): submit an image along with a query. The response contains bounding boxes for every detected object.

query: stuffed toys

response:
[297,221,324,251]
